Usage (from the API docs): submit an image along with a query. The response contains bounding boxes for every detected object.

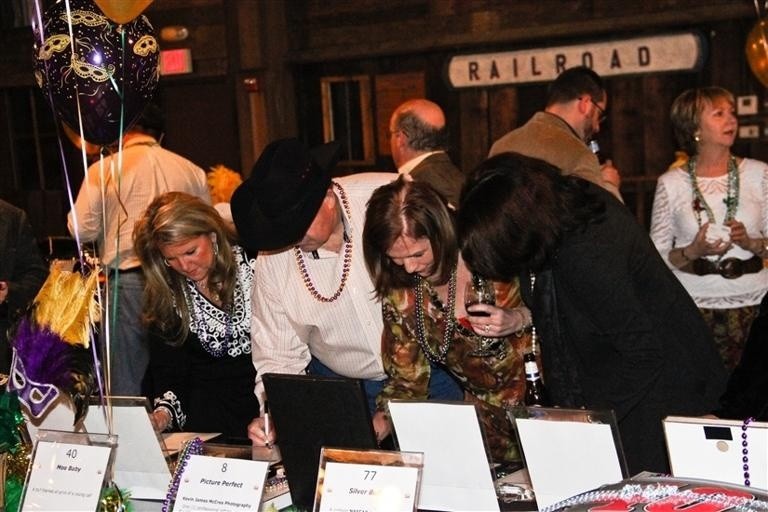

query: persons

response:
[389,99,468,210]
[362,176,545,462]
[488,66,625,205]
[67,106,212,407]
[231,138,464,450]
[1,197,49,430]
[648,86,768,385]
[455,151,727,472]
[131,191,256,453]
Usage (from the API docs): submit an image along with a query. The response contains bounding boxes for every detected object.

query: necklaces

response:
[195,276,220,301]
[294,182,353,302]
[414,267,457,363]
[688,151,739,264]
[530,266,537,356]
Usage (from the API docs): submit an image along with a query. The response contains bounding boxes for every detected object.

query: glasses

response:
[385,130,411,141]
[578,96,607,121]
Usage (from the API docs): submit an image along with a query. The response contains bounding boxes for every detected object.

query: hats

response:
[229,139,333,251]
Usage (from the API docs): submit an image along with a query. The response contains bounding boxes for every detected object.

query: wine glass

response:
[463,280,498,359]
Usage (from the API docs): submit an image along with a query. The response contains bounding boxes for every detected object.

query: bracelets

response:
[514,308,526,338]
[584,414,592,423]
[681,248,691,262]
[759,239,768,259]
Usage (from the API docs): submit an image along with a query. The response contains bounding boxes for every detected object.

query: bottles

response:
[518,354,547,418]
[586,134,607,168]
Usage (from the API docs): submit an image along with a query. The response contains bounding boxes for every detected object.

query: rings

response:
[485,324,490,334]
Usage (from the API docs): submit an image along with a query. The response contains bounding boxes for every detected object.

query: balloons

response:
[93,0,155,25]
[744,17,768,89]
[30,0,161,147]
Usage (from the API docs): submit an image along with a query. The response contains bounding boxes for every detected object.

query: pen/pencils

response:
[263,399,269,447]
